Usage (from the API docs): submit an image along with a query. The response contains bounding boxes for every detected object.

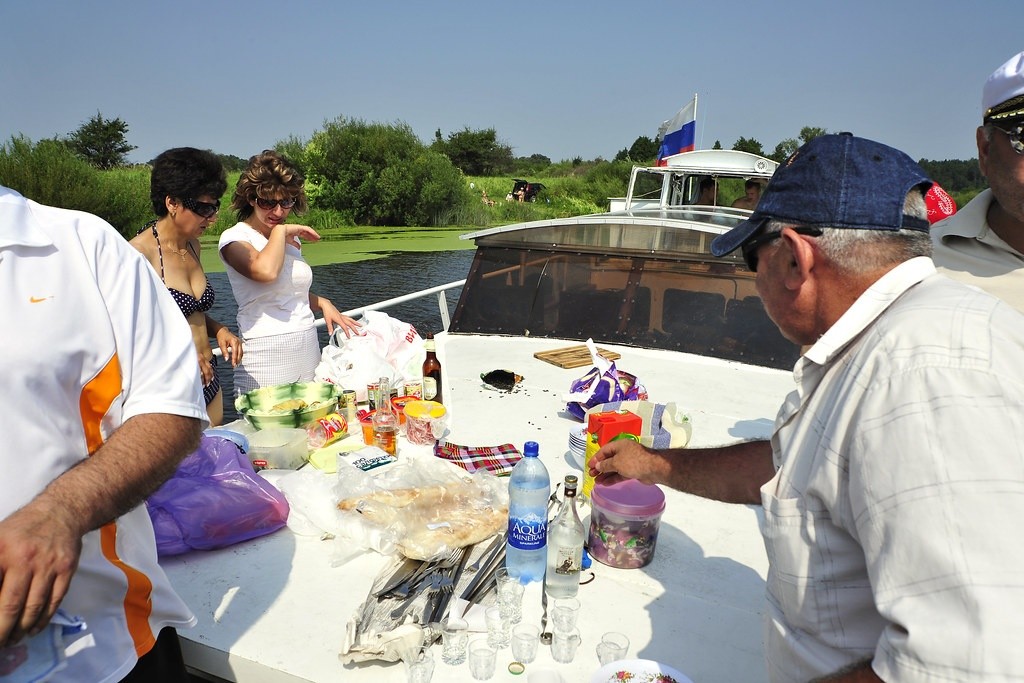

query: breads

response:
[330,474,512,564]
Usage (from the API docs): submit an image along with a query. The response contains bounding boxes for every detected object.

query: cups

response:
[396,568,630,683]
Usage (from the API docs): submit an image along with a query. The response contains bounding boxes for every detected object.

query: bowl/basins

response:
[235,380,339,430]
[203,429,250,453]
[569,422,589,474]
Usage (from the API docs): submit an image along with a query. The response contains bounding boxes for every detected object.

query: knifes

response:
[372,530,511,624]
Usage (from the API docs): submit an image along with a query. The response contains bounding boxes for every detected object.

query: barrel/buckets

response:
[588,473,666,570]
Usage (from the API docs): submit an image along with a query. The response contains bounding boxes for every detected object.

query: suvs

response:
[510,177,551,204]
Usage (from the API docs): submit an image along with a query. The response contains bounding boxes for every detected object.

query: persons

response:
[123,145,242,430]
[469,180,526,209]
[731,179,763,211]
[583,128,1023,683]
[690,177,724,207]
[216,149,366,422]
[919,51,1024,316]
[0,186,215,683]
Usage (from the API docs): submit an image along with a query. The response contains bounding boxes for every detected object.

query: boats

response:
[154,91,871,683]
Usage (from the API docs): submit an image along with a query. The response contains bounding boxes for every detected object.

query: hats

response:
[710,132,938,258]
[982,50,1024,134]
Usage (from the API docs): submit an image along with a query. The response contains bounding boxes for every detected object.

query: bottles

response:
[506,441,548,584]
[547,475,586,598]
[373,376,397,457]
[421,331,443,406]
[296,407,363,447]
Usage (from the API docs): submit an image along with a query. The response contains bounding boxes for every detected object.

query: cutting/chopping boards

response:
[533,345,621,369]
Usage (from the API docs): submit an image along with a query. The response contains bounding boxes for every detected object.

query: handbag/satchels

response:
[316,310,424,403]
[336,451,509,561]
[146,434,292,560]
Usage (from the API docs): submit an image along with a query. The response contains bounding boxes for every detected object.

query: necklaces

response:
[155,221,189,262]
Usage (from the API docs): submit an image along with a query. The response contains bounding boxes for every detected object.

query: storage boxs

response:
[248,427,309,471]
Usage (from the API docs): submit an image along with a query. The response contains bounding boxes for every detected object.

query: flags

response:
[654,98,697,167]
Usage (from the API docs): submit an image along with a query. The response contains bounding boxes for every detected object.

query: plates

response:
[589,658,696,683]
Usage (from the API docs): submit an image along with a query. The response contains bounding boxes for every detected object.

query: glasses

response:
[994,123,1024,153]
[744,225,823,270]
[178,197,220,218]
[249,192,296,208]
